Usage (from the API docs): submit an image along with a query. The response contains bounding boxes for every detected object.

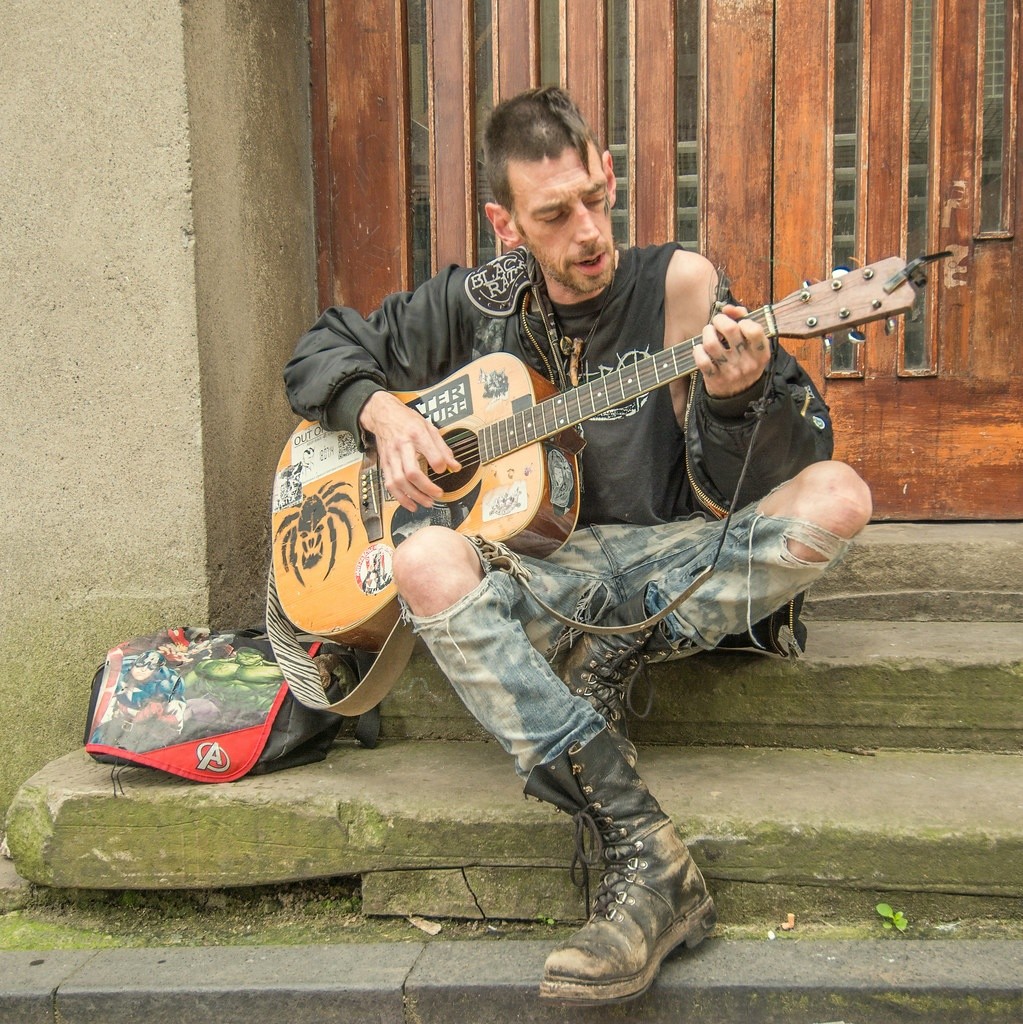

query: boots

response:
[522,727,718,1006]
[556,580,688,768]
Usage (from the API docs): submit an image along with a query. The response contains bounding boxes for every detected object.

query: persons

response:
[283,86,872,1006]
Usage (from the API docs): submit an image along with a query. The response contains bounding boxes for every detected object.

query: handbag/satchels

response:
[83,619,382,785]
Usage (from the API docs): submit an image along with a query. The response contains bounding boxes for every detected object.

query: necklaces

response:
[557,262,615,388]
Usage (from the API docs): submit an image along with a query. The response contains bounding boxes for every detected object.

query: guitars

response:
[271,257,918,654]
